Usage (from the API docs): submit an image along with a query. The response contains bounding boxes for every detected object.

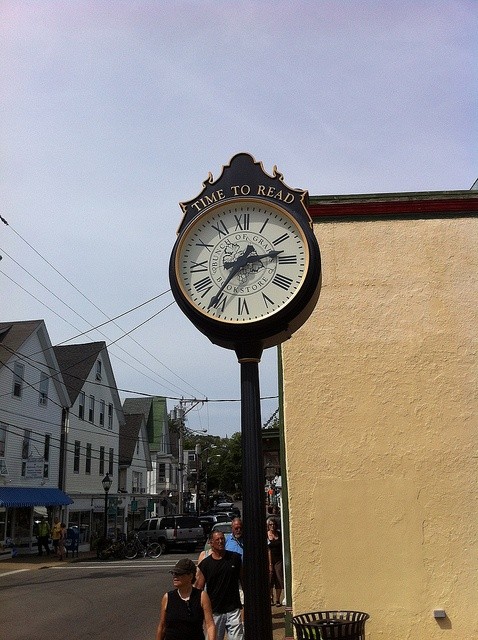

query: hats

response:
[168,558,196,574]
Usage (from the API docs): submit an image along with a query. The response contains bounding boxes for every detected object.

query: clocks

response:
[168,151,322,363]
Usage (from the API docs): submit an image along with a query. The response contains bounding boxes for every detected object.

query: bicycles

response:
[97,532,125,560]
[125,530,162,560]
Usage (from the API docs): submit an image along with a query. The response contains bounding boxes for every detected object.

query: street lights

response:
[101,474,113,557]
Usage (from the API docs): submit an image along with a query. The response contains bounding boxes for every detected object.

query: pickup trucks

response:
[130,516,203,551]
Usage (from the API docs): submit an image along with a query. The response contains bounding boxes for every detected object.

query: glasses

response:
[171,572,187,576]
[185,600,192,616]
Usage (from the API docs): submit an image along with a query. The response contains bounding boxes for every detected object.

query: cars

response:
[204,522,233,553]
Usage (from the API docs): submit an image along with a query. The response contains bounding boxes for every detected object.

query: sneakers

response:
[271,600,274,606]
[276,601,281,606]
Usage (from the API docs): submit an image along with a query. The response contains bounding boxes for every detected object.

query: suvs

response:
[213,511,236,517]
[199,515,229,523]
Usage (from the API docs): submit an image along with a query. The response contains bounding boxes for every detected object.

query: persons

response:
[195,530,245,640]
[224,518,245,633]
[36,516,50,556]
[51,517,66,560]
[266,517,283,607]
[156,558,216,640]
